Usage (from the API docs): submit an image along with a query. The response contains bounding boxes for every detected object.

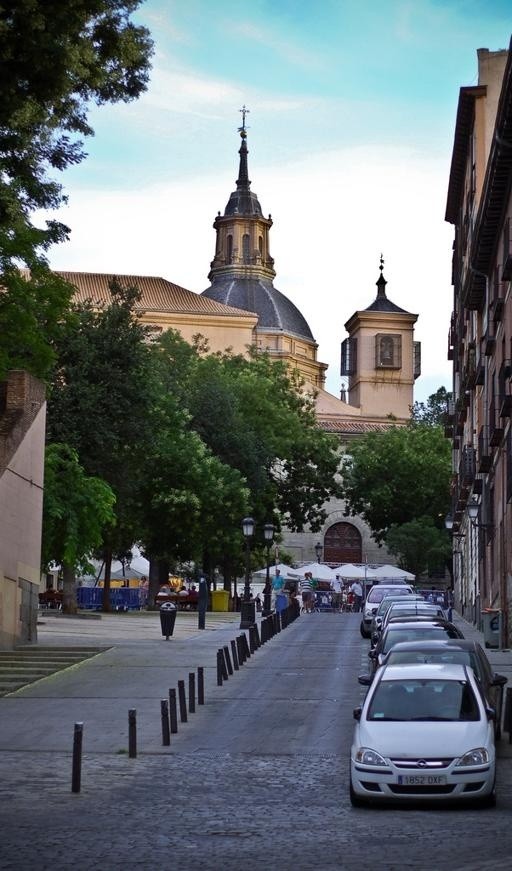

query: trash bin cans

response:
[481,607,500,649]
[159,602,177,641]
[211,589,230,612]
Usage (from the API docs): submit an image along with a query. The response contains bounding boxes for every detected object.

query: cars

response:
[349,585,508,808]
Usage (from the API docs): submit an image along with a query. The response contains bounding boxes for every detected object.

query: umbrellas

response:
[255,560,417,590]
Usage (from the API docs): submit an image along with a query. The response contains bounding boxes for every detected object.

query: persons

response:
[156,583,200,612]
[137,575,150,613]
[419,586,454,609]
[241,569,364,613]
[44,584,60,609]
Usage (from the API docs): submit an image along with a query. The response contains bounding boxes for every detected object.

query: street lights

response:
[261,521,278,618]
[239,517,256,630]
[118,548,133,588]
[314,542,323,565]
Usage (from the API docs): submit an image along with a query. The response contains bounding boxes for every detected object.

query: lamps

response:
[463,492,499,544]
[440,512,467,543]
